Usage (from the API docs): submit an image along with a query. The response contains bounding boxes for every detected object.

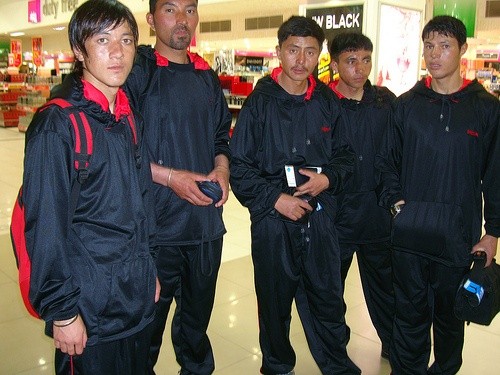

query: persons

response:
[20,0,500,375]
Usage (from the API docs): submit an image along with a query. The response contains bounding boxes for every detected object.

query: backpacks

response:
[9,98,143,320]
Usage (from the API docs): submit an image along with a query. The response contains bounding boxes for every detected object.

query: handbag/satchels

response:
[451,251,500,326]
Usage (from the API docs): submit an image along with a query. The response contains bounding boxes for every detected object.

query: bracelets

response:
[52,314,79,327]
[167,168,173,188]
